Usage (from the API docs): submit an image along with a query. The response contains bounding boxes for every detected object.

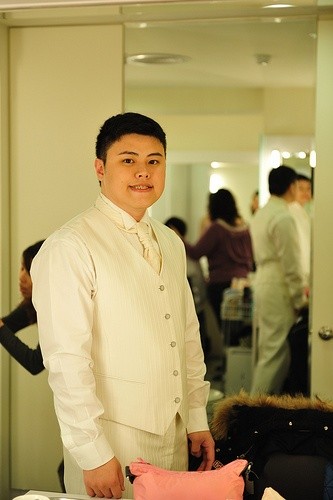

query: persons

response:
[30,111,215,500]
[0,238,66,491]
[164,165,314,397]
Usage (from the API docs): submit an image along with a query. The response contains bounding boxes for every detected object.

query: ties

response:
[95,194,162,276]
